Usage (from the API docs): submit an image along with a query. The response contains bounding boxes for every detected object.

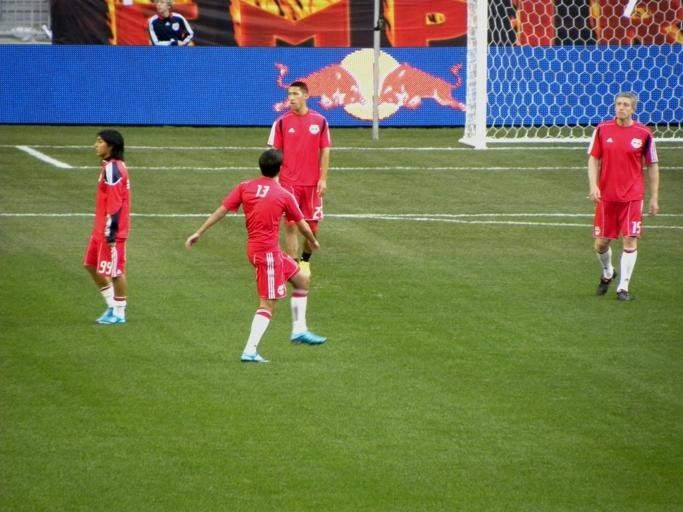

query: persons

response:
[80,128,134,326]
[264,79,331,280]
[582,92,660,301]
[183,148,329,367]
[144,0,196,46]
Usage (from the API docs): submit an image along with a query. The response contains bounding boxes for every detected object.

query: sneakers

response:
[290,331,329,344]
[240,352,270,365]
[596,266,617,295]
[94,306,127,324]
[616,289,632,302]
[298,261,313,277]
